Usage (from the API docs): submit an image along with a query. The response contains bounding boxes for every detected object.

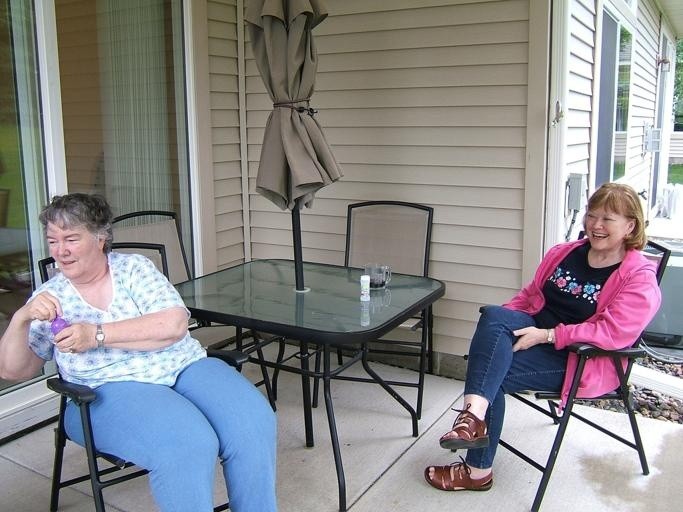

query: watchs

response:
[547,329,552,344]
[94,324,105,348]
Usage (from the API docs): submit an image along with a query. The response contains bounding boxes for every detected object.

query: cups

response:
[363,262,391,290]
[368,289,391,312]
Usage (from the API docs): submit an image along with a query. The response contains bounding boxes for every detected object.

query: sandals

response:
[425,456,493,491]
[440,404,490,449]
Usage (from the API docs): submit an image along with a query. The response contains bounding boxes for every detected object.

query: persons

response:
[0,192,281,510]
[427,181,662,493]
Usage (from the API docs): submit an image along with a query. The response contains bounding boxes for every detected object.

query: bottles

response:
[359,274,370,302]
[48,317,69,337]
[360,302,370,327]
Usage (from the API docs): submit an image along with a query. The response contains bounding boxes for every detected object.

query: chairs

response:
[30,243,249,507]
[108,212,278,416]
[451,232,672,508]
[344,201,437,377]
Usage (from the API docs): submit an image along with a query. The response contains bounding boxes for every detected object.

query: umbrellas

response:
[242,0,343,449]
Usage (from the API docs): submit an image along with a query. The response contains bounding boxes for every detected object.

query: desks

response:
[167,259,446,508]
